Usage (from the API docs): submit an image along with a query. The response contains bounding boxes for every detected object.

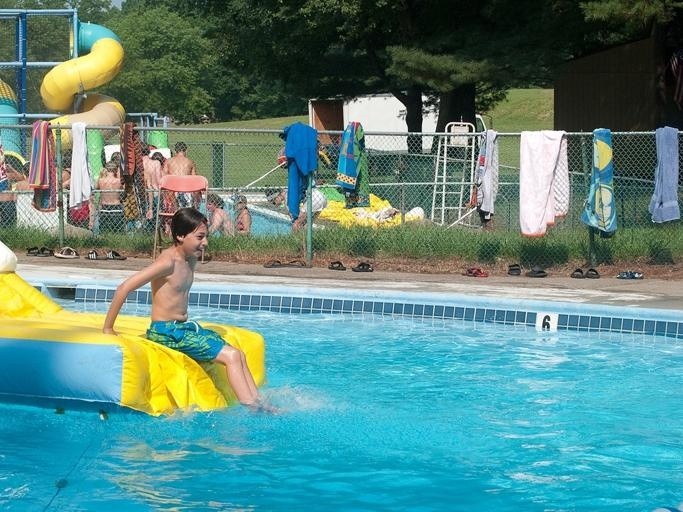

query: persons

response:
[263,185,327,232]
[101,208,284,412]
[12,141,251,239]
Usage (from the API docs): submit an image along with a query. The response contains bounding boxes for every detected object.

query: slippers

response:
[36,247,55,257]
[262,258,283,268]
[526,266,548,278]
[585,268,600,279]
[106,250,128,260]
[506,263,522,276]
[283,259,313,268]
[53,247,80,259]
[85,250,109,261]
[570,268,584,278]
[26,246,39,256]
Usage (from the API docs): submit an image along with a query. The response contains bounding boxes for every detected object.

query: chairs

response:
[153,174,212,264]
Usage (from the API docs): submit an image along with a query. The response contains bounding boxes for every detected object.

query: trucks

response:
[307,90,493,179]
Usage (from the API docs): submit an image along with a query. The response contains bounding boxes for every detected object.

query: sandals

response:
[465,266,489,277]
[328,260,347,271]
[352,262,374,272]
[617,270,645,280]
